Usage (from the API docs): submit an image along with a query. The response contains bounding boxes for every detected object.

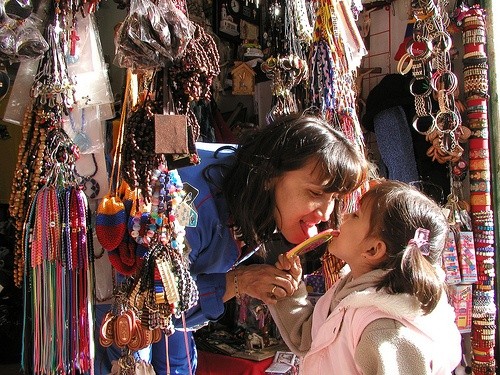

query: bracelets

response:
[396,0,497,375]
[233,267,243,300]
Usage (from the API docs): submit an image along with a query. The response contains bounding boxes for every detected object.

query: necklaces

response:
[0,0,219,375]
[262,0,370,160]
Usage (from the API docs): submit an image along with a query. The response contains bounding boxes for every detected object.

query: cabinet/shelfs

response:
[196,350,273,375]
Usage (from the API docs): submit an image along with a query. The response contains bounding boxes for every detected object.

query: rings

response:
[271,285,277,293]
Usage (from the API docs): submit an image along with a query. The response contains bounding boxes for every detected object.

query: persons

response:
[93,113,368,375]
[365,18,452,209]
[266,177,463,375]
